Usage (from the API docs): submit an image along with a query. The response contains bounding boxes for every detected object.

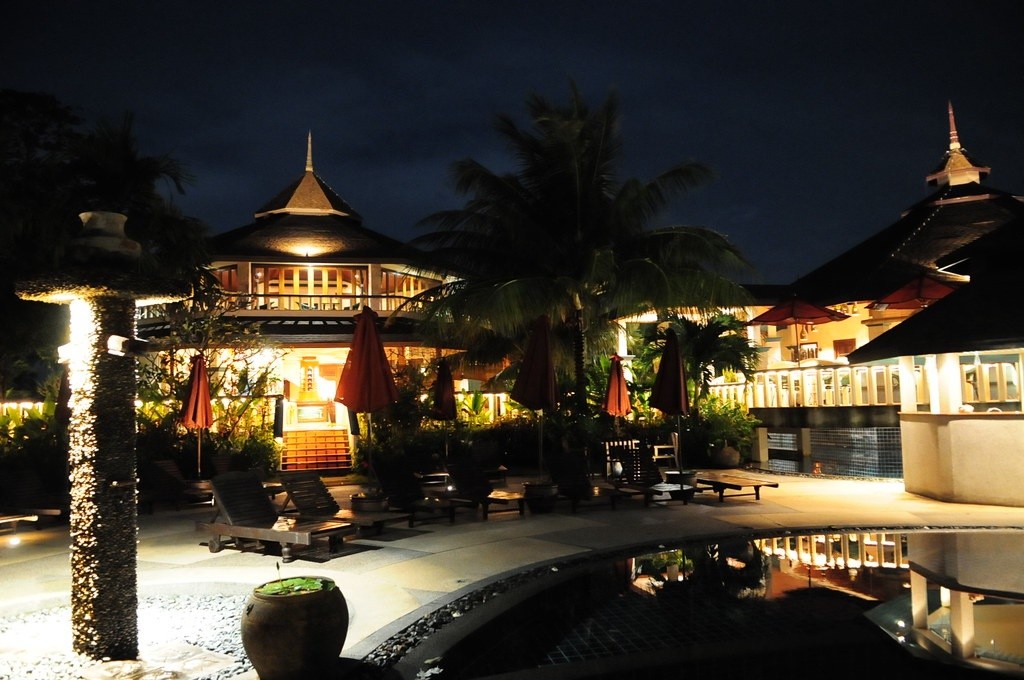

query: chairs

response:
[277,471,413,546]
[190,478,357,564]
[255,302,275,310]
[364,451,466,529]
[696,474,779,502]
[652,432,679,468]
[445,464,543,522]
[344,303,360,310]
[295,301,316,310]
[548,449,694,513]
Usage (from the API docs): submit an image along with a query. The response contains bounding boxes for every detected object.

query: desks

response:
[228,301,252,310]
[322,303,340,310]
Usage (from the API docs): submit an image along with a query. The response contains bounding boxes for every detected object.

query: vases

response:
[665,470,698,501]
[348,492,392,533]
[522,480,557,513]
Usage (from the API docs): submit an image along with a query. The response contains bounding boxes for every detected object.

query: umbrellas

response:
[334,305,403,497]
[744,293,851,367]
[601,353,631,439]
[428,357,458,456]
[648,328,690,490]
[511,314,558,481]
[177,353,214,481]
[865,275,962,310]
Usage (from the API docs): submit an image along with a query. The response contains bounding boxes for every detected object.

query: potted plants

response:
[241,574,350,680]
